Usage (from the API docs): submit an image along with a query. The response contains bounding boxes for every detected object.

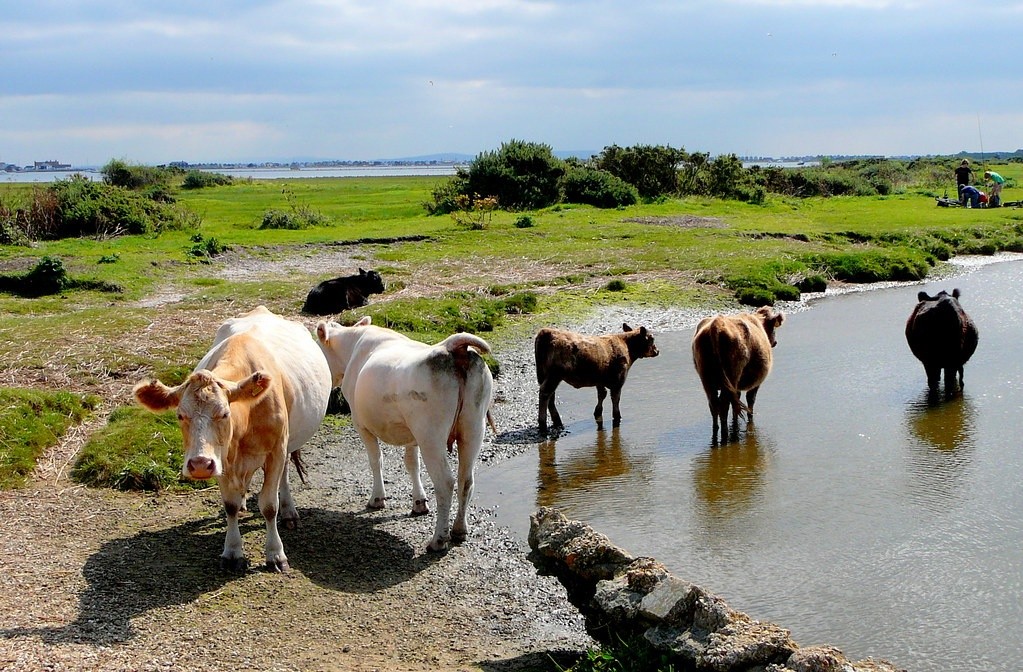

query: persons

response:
[984,171,1005,206]
[959,184,981,208]
[978,192,988,208]
[954,159,975,202]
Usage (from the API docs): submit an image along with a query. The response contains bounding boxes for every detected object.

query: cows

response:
[692,305,784,438]
[905,287,979,392]
[314,315,493,556]
[301,267,385,316]
[131,306,332,573]
[534,322,660,434]
